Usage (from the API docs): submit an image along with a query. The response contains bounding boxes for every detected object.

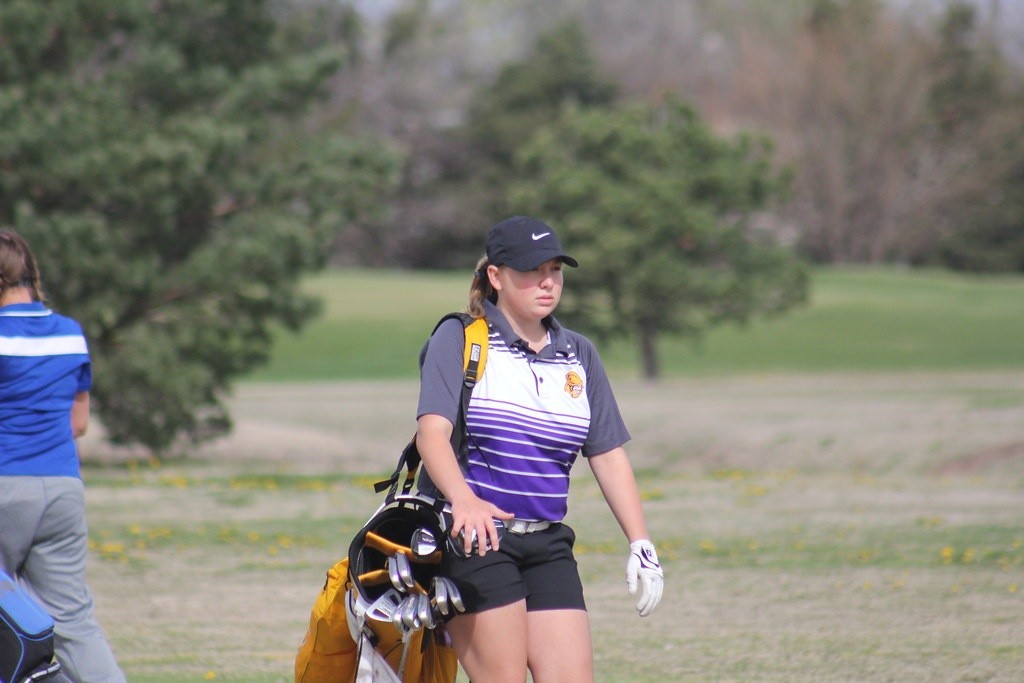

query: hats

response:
[486,216,578,273]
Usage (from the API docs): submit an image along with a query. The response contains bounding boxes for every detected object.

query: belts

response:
[419,494,550,535]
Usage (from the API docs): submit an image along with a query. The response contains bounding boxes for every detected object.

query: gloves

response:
[626,540,664,617]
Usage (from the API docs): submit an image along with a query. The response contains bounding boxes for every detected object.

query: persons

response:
[0,228,127,683]
[414,214,664,683]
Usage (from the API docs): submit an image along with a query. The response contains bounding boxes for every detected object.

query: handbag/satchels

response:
[294,495,458,683]
